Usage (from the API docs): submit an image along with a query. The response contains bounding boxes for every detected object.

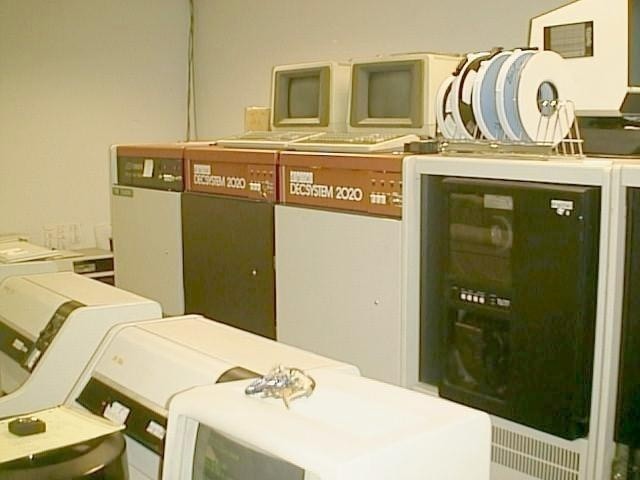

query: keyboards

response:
[216,129,326,149]
[287,130,421,154]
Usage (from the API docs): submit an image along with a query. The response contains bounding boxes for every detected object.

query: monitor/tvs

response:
[346,51,466,140]
[270,60,351,133]
